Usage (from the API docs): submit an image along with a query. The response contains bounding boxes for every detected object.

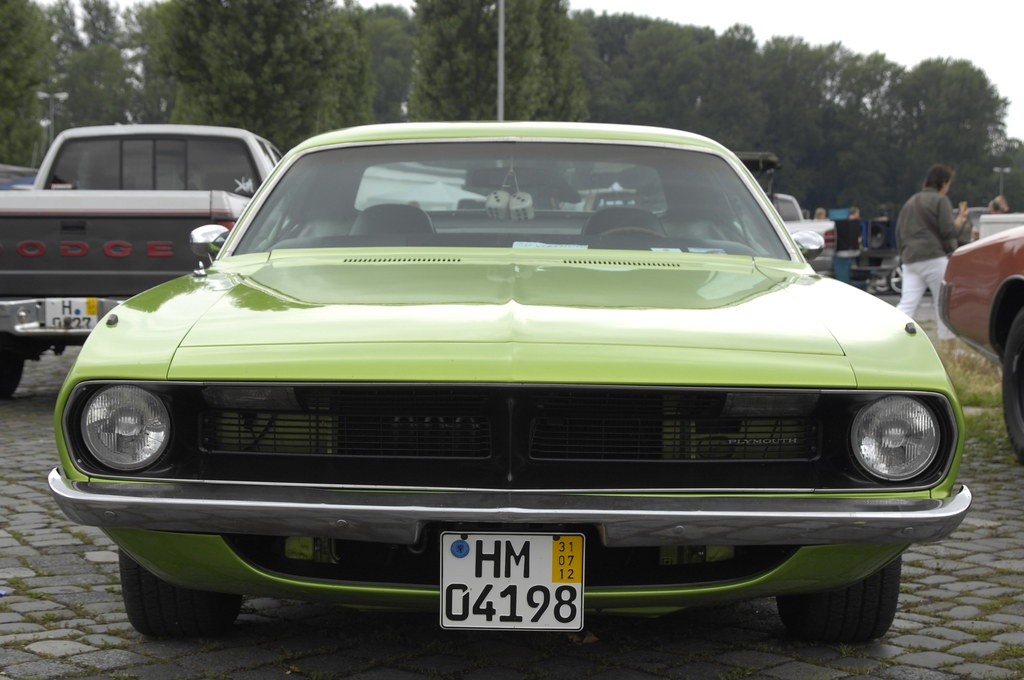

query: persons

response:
[987,195,1023,215]
[547,162,665,210]
[894,166,974,350]
[810,207,862,284]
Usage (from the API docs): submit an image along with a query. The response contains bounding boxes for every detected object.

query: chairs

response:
[348,204,436,237]
[577,206,666,237]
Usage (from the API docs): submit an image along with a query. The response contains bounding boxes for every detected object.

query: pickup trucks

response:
[0,114,289,408]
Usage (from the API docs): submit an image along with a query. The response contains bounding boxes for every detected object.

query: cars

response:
[366,150,1024,463]
[49,124,971,647]
[0,163,64,192]
